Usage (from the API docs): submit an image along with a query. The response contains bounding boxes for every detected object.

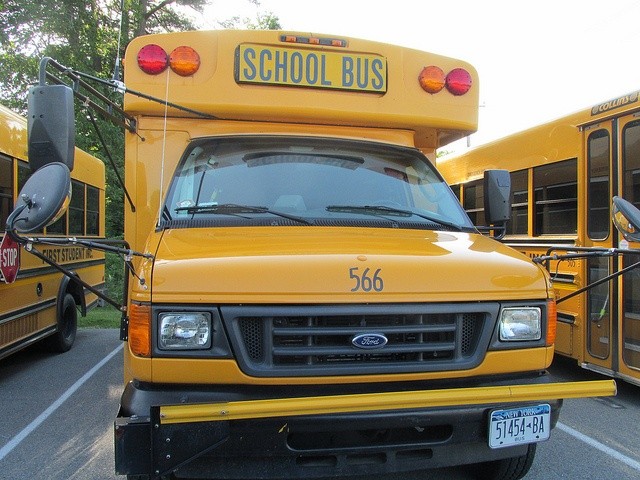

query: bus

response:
[3,27,640,478]
[0,104,107,362]
[438,89,640,388]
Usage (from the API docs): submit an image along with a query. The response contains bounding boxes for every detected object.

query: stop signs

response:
[0,231,21,285]
